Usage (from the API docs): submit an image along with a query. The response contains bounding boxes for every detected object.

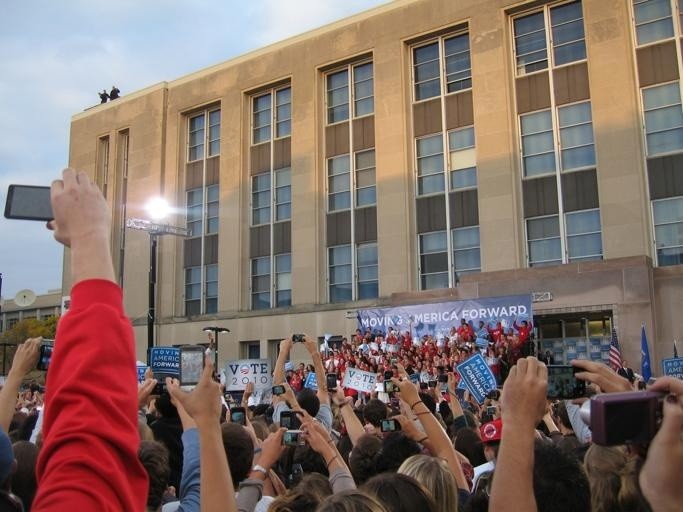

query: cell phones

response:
[326,373,338,393]
[4,185,55,221]
[179,345,204,390]
[542,364,588,399]
[292,333,306,343]
[376,357,501,433]
[281,431,306,446]
[230,406,246,424]
[272,385,286,395]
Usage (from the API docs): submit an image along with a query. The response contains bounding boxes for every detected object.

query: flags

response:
[641,329,652,383]
[609,330,623,371]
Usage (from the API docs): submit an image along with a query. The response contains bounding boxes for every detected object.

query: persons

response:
[97,89,109,104]
[22,165,154,511]
[108,85,120,100]
[352,318,532,358]
[0,337,682,511]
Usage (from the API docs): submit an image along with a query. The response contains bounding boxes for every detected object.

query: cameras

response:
[37,339,54,371]
[150,383,169,395]
[280,410,304,431]
[579,392,668,448]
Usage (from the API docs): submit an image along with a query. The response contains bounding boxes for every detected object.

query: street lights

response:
[125,192,196,381]
[201,324,230,384]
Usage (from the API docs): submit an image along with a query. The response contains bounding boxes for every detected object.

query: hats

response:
[478,417,502,443]
